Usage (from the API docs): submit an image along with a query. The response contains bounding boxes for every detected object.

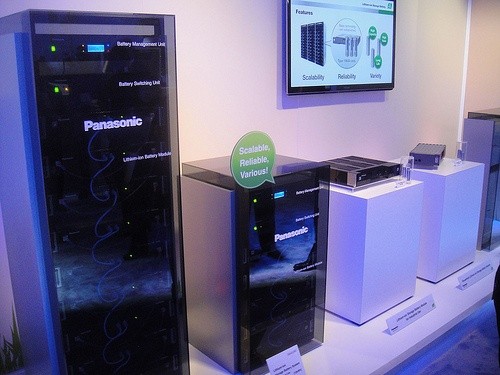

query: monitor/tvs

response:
[286,0,396,96]
